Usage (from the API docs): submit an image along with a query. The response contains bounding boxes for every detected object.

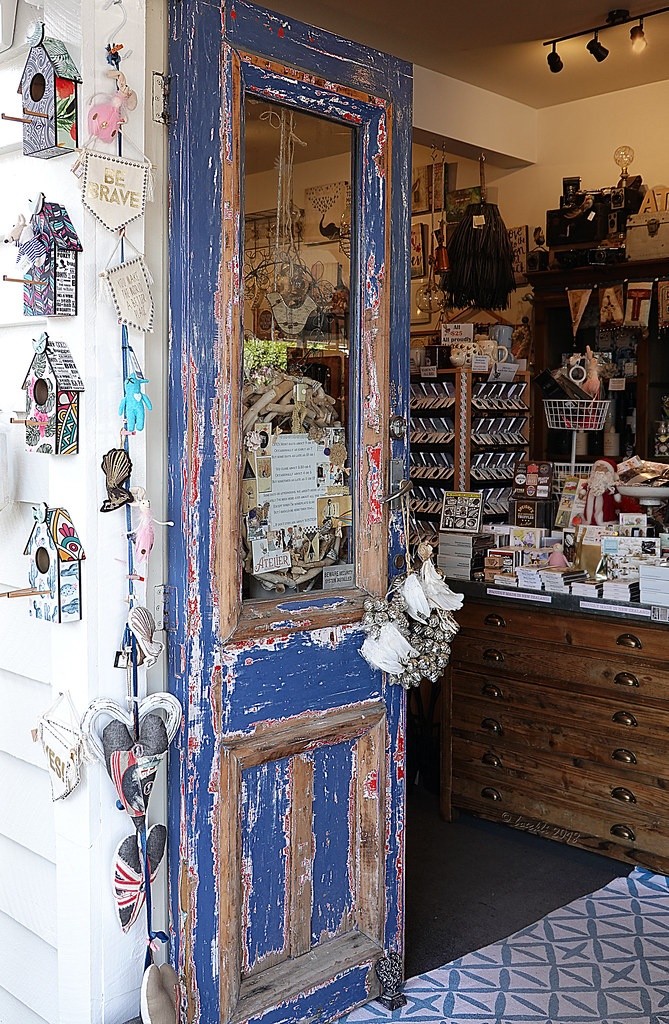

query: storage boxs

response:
[625,211,669,262]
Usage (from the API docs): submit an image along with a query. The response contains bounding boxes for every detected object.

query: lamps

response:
[586,32,609,63]
[629,18,648,53]
[546,43,563,73]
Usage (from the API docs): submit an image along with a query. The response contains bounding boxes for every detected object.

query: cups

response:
[489,325,513,346]
[478,339,508,367]
[424,345,451,368]
[498,349,514,363]
[452,341,484,369]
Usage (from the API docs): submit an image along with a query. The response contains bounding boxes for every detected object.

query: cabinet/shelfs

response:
[410,259,669,876]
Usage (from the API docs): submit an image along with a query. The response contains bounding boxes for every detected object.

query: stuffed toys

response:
[125,487,173,580]
[81,70,131,151]
[119,374,152,432]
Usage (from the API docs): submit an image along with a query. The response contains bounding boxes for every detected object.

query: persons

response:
[578,458,621,526]
[544,543,572,568]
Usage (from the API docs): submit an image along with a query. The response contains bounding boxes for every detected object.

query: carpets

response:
[326,866,669,1024]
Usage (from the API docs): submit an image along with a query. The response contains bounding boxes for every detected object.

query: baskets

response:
[553,462,595,497]
[539,396,611,432]
